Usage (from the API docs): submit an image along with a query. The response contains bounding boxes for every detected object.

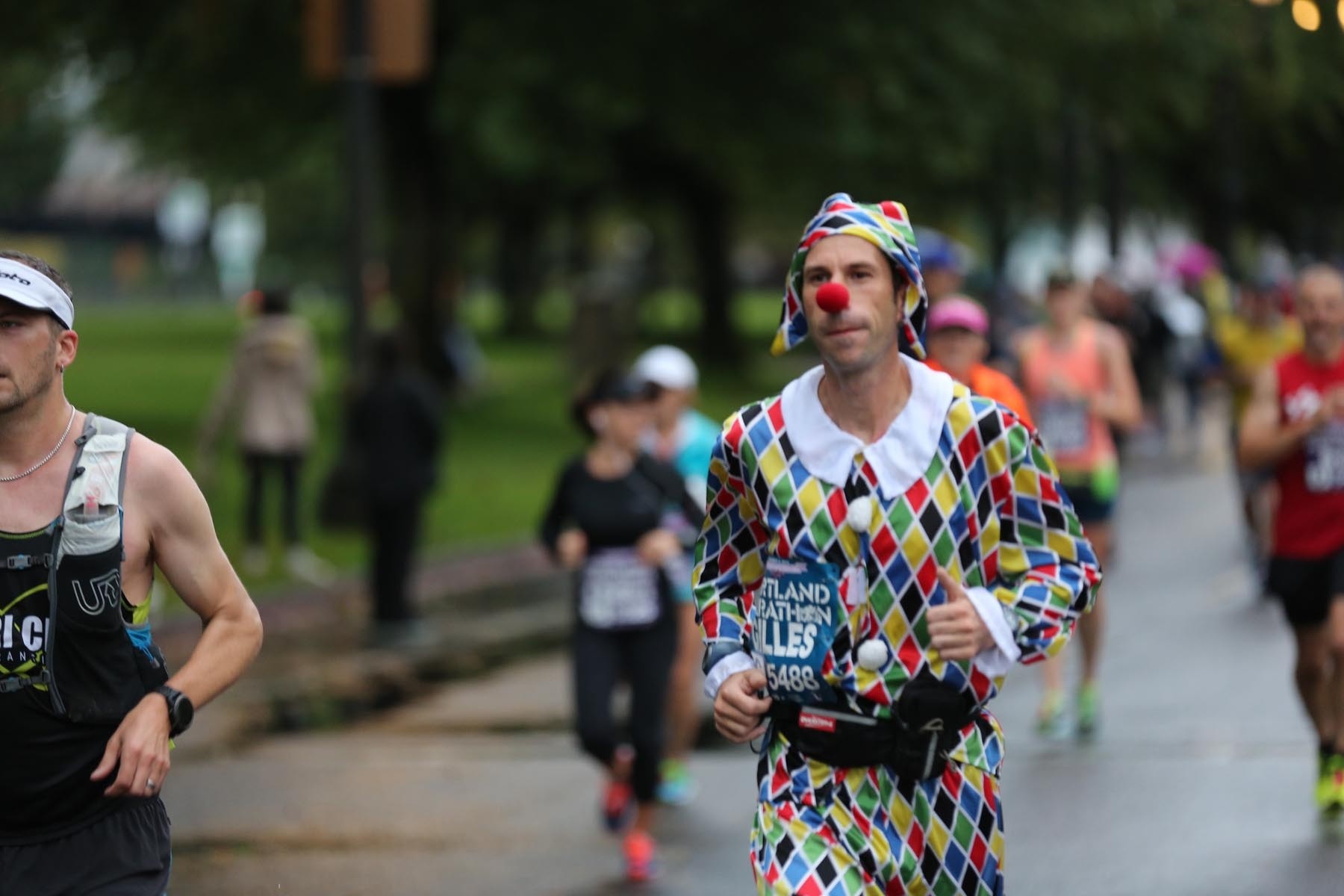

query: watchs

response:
[150,685,195,740]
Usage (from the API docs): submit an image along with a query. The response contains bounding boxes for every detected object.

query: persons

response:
[191,282,471,647]
[535,226,1344,896]
[0,251,265,896]
[693,192,1104,896]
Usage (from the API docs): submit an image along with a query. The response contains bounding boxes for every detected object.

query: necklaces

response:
[0,404,76,483]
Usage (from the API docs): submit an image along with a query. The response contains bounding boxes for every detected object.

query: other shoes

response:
[1032,689,1067,738]
[1076,684,1102,734]
[616,830,667,883]
[602,780,633,832]
[1316,751,1344,815]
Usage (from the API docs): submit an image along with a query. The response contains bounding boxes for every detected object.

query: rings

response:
[146,779,157,790]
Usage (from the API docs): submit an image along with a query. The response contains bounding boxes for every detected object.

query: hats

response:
[768,192,928,362]
[927,293,988,336]
[632,344,698,389]
[0,257,74,330]
[915,230,952,269]
